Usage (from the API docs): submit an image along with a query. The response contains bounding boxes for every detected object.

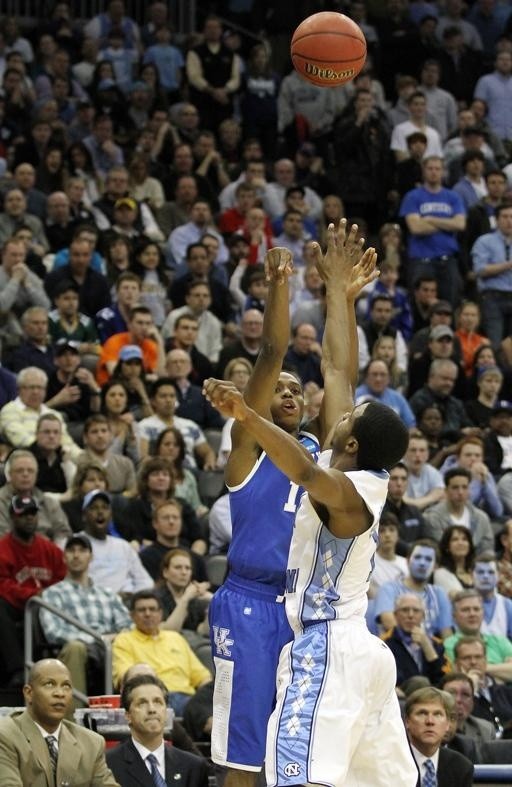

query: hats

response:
[114,198,137,210]
[9,496,39,514]
[67,536,90,549]
[54,338,79,353]
[429,325,453,340]
[119,345,143,361]
[82,489,111,509]
[429,302,452,314]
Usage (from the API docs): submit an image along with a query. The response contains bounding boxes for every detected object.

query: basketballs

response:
[291,12,367,88]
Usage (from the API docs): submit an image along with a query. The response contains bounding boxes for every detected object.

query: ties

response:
[422,760,438,787]
[45,736,58,780]
[147,755,167,787]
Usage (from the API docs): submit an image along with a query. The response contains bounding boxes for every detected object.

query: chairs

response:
[481,740,512,764]
[198,471,224,497]
[207,557,227,585]
[205,431,222,453]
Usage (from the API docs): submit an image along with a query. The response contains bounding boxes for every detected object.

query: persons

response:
[206,246,383,787]
[1,2,510,762]
[201,218,418,787]
[401,687,473,787]
[0,658,120,787]
[104,674,209,787]
